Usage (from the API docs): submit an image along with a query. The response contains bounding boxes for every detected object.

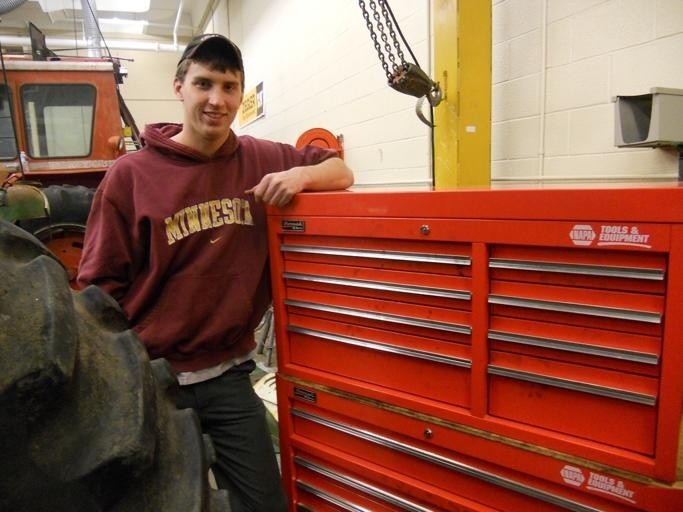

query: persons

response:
[76,33,354,512]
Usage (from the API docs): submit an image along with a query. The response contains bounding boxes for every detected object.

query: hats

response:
[176,32,244,78]
